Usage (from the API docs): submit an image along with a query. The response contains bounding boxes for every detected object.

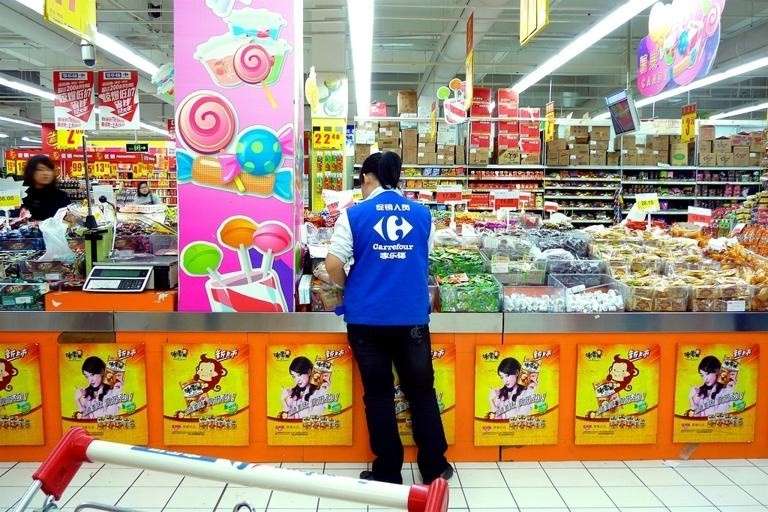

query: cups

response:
[443,97,467,125]
[204,266,290,314]
[192,7,293,90]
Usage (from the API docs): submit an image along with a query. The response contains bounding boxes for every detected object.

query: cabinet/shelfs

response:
[402,166,768,228]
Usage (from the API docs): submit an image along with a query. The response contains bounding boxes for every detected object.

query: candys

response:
[438,87,450,101]
[233,42,278,110]
[176,151,294,204]
[178,93,246,193]
[184,244,226,288]
[218,127,294,187]
[252,223,291,278]
[451,80,461,100]
[460,82,466,96]
[220,218,255,283]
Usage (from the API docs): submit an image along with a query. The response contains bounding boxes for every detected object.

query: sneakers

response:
[360,470,402,484]
[424,463,453,485]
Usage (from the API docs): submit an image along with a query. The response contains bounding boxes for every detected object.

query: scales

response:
[82,257,179,292]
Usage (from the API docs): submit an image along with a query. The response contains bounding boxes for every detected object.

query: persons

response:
[691,355,740,416]
[489,357,539,419]
[73,356,131,418]
[11,154,69,222]
[326,152,453,485]
[280,357,333,419]
[131,181,163,206]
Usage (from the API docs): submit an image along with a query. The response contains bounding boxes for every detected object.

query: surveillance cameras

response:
[81,45,96,66]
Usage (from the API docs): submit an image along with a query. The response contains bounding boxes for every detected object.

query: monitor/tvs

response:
[605,89,640,137]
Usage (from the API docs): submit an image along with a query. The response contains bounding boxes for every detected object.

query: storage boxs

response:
[355,86,464,167]
[466,87,543,167]
[539,123,768,167]
